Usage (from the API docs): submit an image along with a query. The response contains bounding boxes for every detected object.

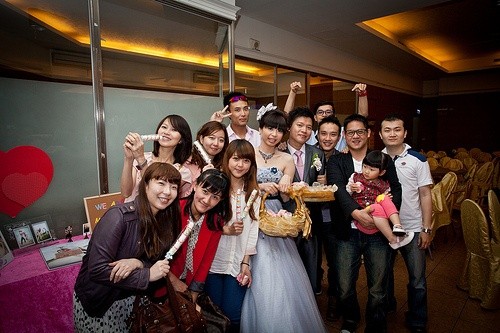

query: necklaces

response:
[259,150,274,163]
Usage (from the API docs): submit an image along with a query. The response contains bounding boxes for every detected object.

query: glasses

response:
[317,110,333,115]
[228,96,248,103]
[347,130,367,136]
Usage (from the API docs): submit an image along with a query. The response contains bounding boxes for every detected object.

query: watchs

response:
[422,228,432,234]
[136,160,147,170]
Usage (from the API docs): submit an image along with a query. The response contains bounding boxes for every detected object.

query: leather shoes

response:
[326,296,341,321]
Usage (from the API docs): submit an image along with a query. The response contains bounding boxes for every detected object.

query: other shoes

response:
[339,320,357,333]
[387,302,396,314]
[389,231,415,250]
[392,224,406,236]
[315,285,323,295]
[411,326,426,333]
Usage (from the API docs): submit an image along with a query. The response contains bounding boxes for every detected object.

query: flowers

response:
[312,153,322,171]
[256,103,277,120]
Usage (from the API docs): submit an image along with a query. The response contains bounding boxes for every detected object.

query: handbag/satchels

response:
[296,197,313,238]
[195,293,232,333]
[126,274,207,333]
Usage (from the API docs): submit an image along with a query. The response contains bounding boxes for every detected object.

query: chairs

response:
[418,147,500,309]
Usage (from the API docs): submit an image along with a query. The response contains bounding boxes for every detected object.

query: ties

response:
[295,151,302,180]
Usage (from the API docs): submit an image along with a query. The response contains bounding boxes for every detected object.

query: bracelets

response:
[240,262,250,267]
[359,90,367,97]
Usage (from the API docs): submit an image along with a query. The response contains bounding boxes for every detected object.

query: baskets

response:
[259,185,306,237]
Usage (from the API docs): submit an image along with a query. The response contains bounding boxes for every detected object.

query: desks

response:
[0,234,96,333]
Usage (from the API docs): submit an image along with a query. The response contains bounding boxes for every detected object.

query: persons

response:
[326,113,402,333]
[185,81,369,333]
[72,162,182,333]
[120,114,201,203]
[167,168,233,303]
[377,116,434,333]
[345,150,415,250]
